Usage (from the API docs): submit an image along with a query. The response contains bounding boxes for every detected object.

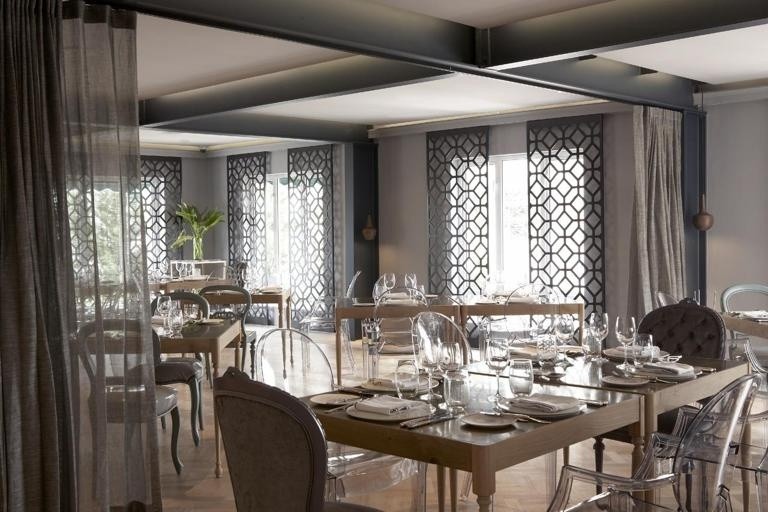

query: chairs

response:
[212,367,379,510]
[369,291,431,379]
[254,328,415,492]
[131,290,210,446]
[198,283,249,374]
[481,285,558,367]
[721,281,767,394]
[661,395,767,512]
[75,318,182,499]
[415,313,470,380]
[169,201,223,259]
[593,299,726,511]
[547,373,759,512]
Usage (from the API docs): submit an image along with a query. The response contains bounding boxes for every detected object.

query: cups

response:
[632,331,654,362]
[509,359,533,398]
[536,335,557,366]
[168,310,183,335]
[443,369,471,414]
[394,359,419,397]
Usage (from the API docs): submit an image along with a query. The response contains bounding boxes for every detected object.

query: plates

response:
[360,376,439,394]
[630,362,699,378]
[500,392,587,418]
[603,375,650,387]
[342,396,433,419]
[463,412,519,429]
[311,392,362,405]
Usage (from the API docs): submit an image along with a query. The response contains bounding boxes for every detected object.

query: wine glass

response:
[555,312,637,370]
[485,336,508,404]
[176,263,193,279]
[382,272,395,302]
[405,272,418,298]
[157,296,171,328]
[435,340,462,407]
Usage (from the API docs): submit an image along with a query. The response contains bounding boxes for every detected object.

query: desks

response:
[718,311,767,340]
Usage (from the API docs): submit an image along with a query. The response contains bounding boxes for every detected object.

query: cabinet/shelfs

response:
[169,259,226,281]
